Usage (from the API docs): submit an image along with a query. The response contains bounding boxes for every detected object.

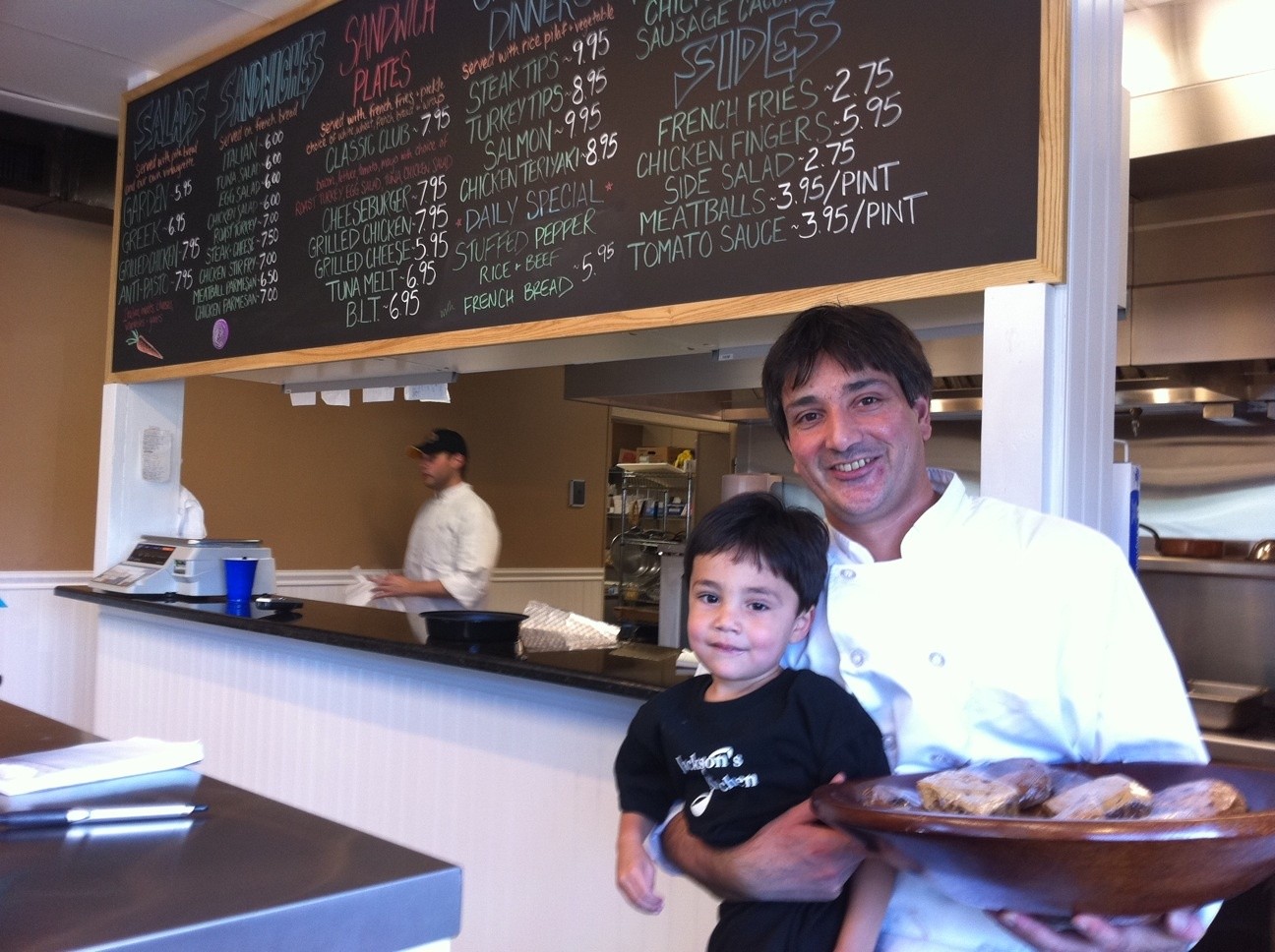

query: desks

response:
[1,701,463,952]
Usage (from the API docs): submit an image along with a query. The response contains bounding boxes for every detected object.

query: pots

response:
[1139,524,1249,559]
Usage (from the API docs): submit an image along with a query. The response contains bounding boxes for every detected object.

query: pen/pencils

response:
[0,802,209,831]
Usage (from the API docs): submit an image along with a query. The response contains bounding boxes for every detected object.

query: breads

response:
[861,758,1246,820]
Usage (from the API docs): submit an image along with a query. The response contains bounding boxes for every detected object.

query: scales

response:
[81,531,279,595]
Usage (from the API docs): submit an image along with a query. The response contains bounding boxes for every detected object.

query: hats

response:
[412,427,468,458]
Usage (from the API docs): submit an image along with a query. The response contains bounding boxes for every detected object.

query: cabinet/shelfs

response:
[617,473,693,607]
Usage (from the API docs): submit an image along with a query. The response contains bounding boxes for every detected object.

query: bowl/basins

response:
[808,757,1274,928]
[418,610,529,644]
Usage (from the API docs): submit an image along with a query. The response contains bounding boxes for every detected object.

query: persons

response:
[648,304,1226,952]
[613,491,898,952]
[367,428,502,613]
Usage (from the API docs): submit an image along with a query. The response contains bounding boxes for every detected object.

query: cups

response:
[225,601,252,618]
[222,558,258,601]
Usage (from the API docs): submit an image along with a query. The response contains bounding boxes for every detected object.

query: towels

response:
[175,482,209,541]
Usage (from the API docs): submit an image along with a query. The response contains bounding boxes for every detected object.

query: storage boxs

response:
[620,446,696,467]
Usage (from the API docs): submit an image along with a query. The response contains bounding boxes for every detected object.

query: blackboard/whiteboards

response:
[103,1,1065,393]
